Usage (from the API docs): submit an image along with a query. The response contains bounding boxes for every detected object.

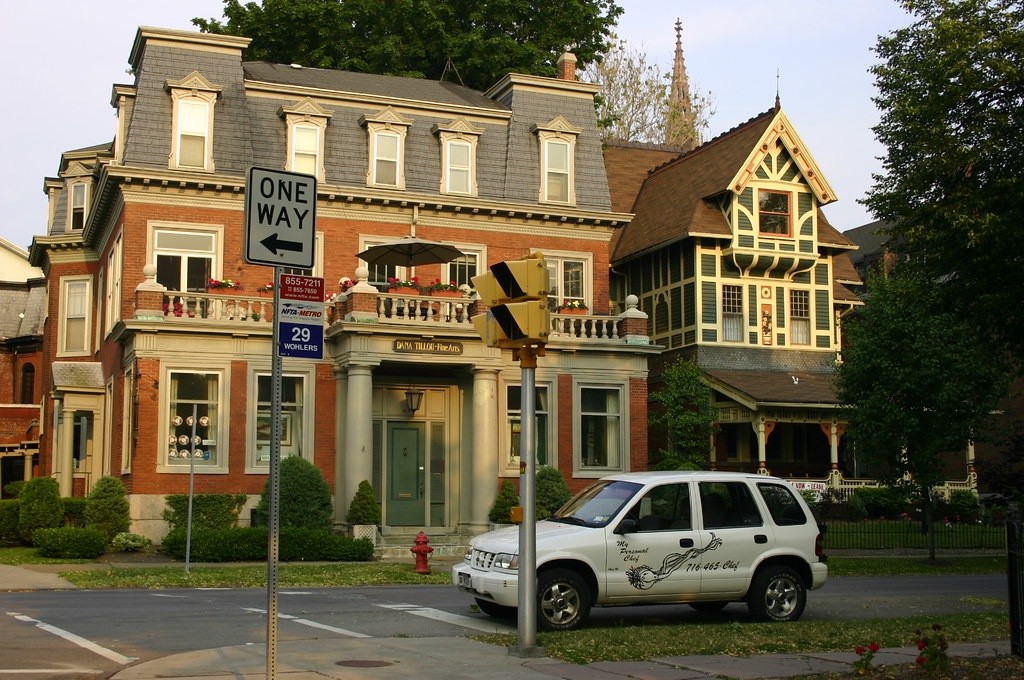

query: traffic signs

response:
[242,166,317,273]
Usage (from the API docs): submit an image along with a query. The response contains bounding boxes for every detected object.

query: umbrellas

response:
[355,236,466,281]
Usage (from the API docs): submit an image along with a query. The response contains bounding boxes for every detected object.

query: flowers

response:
[254,281,275,292]
[556,299,588,310]
[205,277,242,290]
[423,277,461,291]
[380,276,420,291]
[339,279,358,290]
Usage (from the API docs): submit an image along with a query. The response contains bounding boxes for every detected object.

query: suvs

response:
[450,467,831,632]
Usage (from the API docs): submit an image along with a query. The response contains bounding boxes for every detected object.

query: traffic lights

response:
[489,258,552,343]
[469,269,524,350]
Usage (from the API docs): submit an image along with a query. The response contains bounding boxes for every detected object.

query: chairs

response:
[672,497,690,528]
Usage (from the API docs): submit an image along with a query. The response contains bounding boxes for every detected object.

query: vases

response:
[388,286,420,295]
[207,287,243,295]
[430,290,462,298]
[559,307,587,315]
[260,290,274,297]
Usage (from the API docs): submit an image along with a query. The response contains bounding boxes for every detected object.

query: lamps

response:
[404,381,425,416]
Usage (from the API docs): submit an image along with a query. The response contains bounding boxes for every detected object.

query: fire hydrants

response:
[410,530,436,574]
